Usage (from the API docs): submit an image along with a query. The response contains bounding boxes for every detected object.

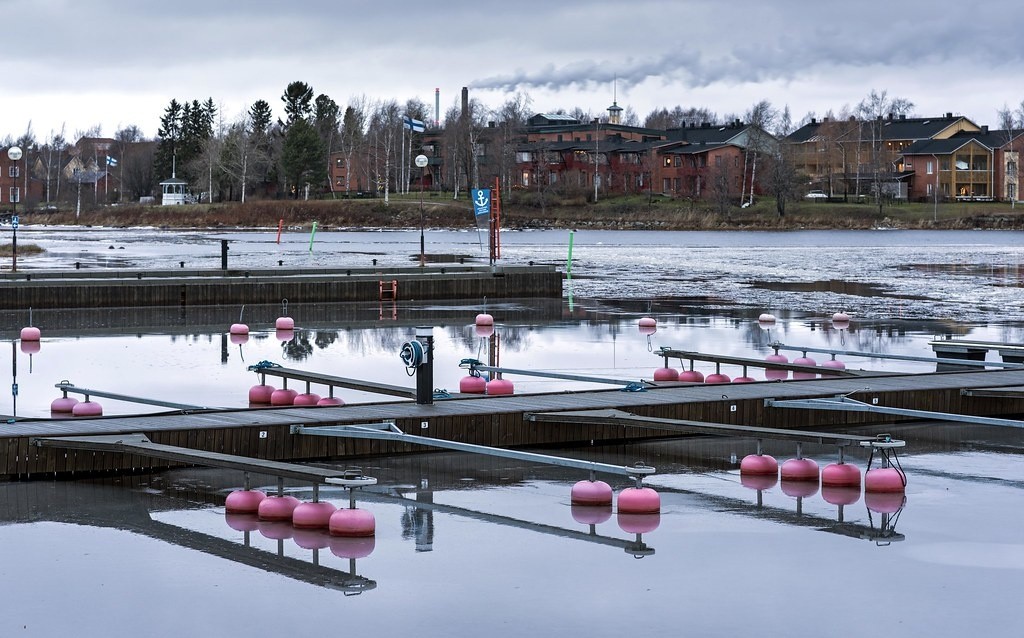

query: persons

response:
[291,186,296,199]
[111,187,118,204]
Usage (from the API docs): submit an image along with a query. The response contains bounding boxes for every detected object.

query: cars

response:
[805,189,827,198]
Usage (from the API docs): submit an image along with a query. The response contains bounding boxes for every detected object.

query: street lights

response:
[415,154,429,264]
[8,146,23,273]
